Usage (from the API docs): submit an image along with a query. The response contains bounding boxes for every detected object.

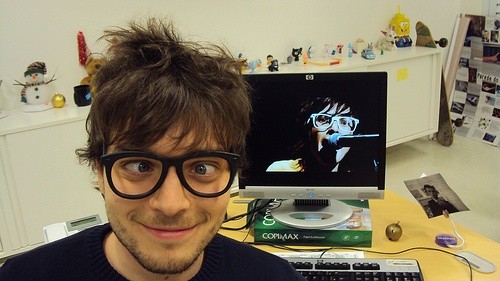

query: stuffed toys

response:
[389,4,413,48]
[80,56,112,104]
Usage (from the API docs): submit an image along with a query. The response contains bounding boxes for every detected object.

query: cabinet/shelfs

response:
[0,45,442,259]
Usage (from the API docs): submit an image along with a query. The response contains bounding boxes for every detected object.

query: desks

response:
[218,190,500,281]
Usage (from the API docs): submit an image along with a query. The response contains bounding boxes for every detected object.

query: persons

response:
[266,94,374,174]
[266,55,273,67]
[481,30,500,64]
[424,184,460,217]
[0,16,309,281]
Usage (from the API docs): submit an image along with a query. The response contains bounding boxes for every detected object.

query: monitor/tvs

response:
[236,72,388,230]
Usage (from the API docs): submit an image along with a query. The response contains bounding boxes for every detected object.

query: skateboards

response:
[416,21,464,147]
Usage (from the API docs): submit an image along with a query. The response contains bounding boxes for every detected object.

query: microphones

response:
[328,133,380,150]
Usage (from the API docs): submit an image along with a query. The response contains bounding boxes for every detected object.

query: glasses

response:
[99,150,243,200]
[306,113,359,133]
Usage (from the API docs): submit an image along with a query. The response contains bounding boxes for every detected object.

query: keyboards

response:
[280,258,424,281]
